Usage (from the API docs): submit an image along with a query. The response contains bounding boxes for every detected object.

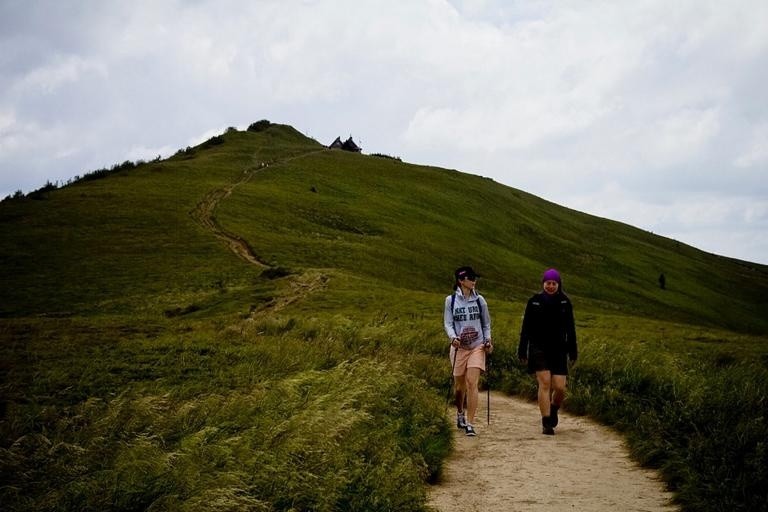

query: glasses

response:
[462,274,476,281]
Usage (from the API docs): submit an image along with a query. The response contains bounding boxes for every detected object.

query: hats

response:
[455,266,480,280]
[543,269,560,284]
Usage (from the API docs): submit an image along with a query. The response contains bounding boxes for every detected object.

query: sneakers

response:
[457,409,467,429]
[465,423,477,437]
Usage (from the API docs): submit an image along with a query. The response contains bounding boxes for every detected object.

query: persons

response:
[517,267,578,434]
[443,266,494,436]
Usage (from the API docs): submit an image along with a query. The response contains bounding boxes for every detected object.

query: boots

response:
[550,404,560,427]
[542,416,554,434]
